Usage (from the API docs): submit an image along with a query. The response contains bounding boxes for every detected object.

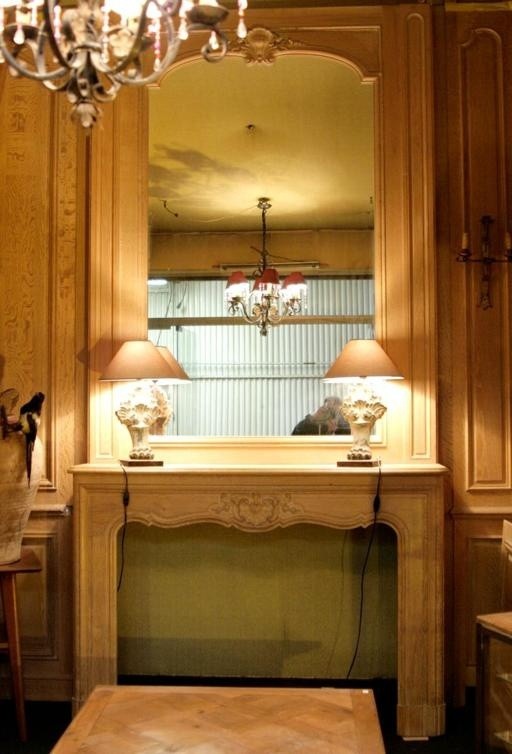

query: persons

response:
[290,396,352,435]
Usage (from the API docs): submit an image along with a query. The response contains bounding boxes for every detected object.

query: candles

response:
[504,231,512,250]
[461,232,470,251]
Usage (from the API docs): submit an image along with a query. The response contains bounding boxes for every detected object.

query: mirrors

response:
[90,6,438,462]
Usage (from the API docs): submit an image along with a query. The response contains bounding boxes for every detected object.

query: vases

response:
[0,416,46,564]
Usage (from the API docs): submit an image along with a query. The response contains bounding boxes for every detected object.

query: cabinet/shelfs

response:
[477,612,512,753]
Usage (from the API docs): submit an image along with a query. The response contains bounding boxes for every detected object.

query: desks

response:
[0,549,43,743]
[49,684,389,753]
[66,463,454,737]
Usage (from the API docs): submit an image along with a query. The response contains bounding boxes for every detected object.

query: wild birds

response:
[1,389,47,490]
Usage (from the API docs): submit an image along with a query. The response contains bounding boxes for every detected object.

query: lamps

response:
[225,197,309,337]
[322,340,406,467]
[146,346,192,437]
[0,1,249,135]
[99,340,179,467]
